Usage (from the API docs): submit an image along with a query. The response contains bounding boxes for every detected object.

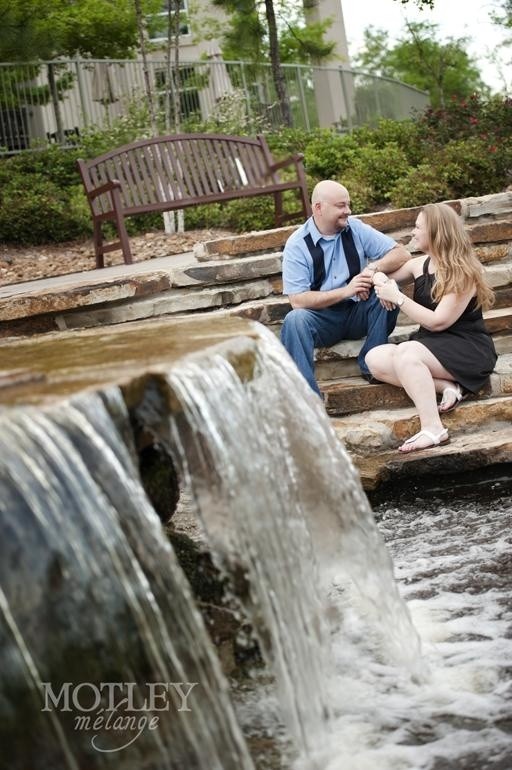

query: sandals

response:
[437,384,463,414]
[399,426,452,454]
[329,300,358,312]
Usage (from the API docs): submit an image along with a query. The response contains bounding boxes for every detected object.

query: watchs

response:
[366,261,379,272]
[396,295,406,306]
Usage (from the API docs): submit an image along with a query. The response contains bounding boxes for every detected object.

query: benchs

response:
[77,130,316,265]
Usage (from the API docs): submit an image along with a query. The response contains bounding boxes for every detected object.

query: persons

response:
[281,180,412,399]
[364,204,498,453]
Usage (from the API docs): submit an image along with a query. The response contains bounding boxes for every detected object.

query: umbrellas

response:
[92,58,119,128]
[208,39,235,101]
